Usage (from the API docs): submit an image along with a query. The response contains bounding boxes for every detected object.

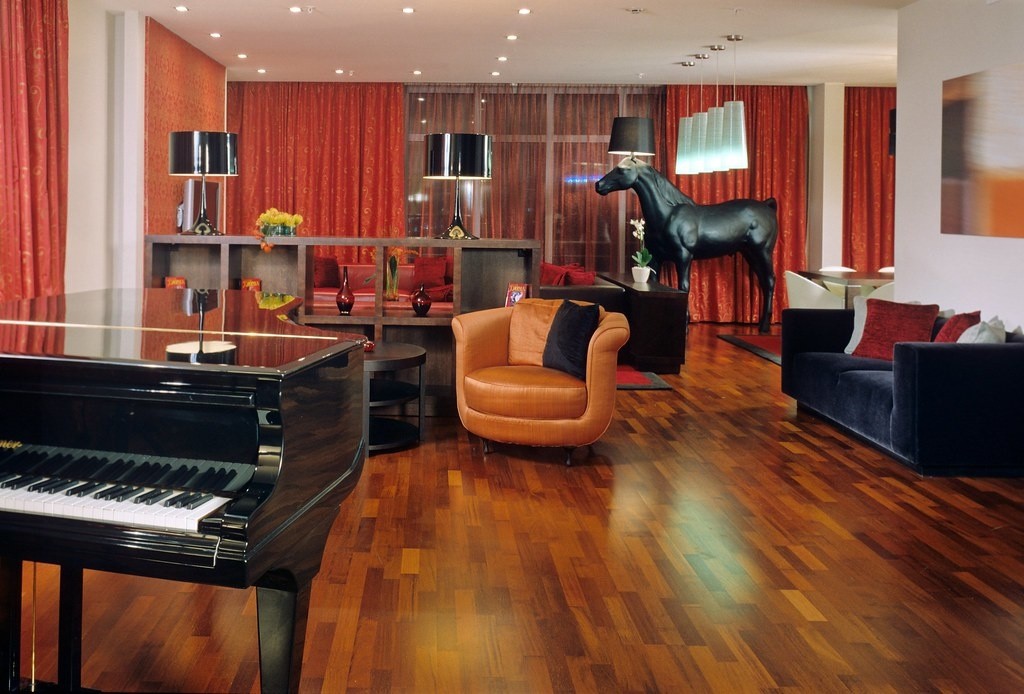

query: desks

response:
[597,271,689,374]
[798,270,894,309]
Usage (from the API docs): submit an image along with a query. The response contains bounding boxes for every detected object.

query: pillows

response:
[843,295,1006,360]
[542,299,599,381]
[540,263,596,286]
[414,256,454,302]
[313,256,341,289]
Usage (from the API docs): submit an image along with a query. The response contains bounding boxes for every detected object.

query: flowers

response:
[362,244,421,301]
[255,207,303,253]
[630,218,652,266]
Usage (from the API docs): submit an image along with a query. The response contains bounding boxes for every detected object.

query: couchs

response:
[783,308,1024,478]
[451,298,630,466]
[313,264,629,366]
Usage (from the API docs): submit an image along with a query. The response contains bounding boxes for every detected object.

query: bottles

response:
[412,283,432,317]
[336,266,355,314]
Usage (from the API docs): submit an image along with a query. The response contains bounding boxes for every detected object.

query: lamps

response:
[423,133,492,240]
[608,117,656,159]
[675,34,748,174]
[168,131,239,236]
[165,289,237,365]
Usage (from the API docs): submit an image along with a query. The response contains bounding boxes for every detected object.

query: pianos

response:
[0,287,368,694]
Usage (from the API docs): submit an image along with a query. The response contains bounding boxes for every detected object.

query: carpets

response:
[717,335,782,365]
[616,359,672,389]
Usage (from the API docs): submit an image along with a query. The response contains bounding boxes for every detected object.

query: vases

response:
[632,266,651,282]
[263,224,296,237]
[385,256,399,301]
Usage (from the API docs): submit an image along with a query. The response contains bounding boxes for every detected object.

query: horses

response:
[594,153,778,334]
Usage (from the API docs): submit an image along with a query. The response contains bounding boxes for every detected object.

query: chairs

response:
[785,266,894,309]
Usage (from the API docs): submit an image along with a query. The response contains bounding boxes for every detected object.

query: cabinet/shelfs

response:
[143,234,540,395]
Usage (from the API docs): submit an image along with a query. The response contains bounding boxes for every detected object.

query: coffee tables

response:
[363,342,427,457]
